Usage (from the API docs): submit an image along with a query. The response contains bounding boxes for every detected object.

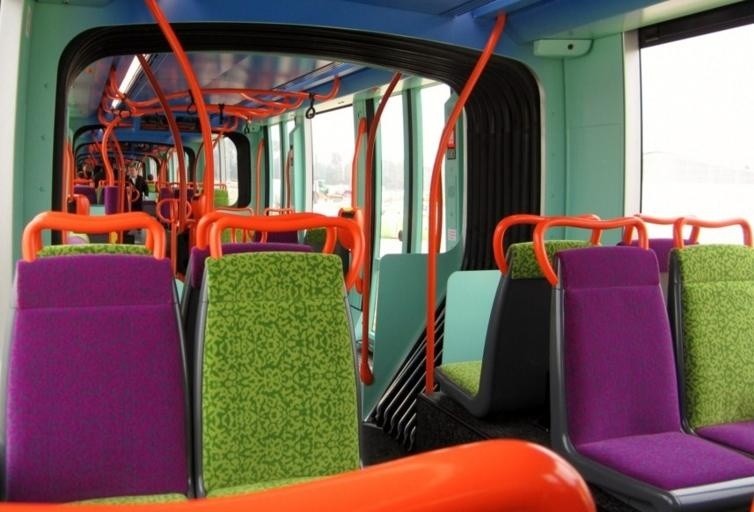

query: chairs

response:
[67,173,354,278]
[2,210,364,510]
[430,211,753,509]
[6,436,598,510]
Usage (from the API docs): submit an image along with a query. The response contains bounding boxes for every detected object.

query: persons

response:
[127,167,148,214]
[147,174,153,181]
